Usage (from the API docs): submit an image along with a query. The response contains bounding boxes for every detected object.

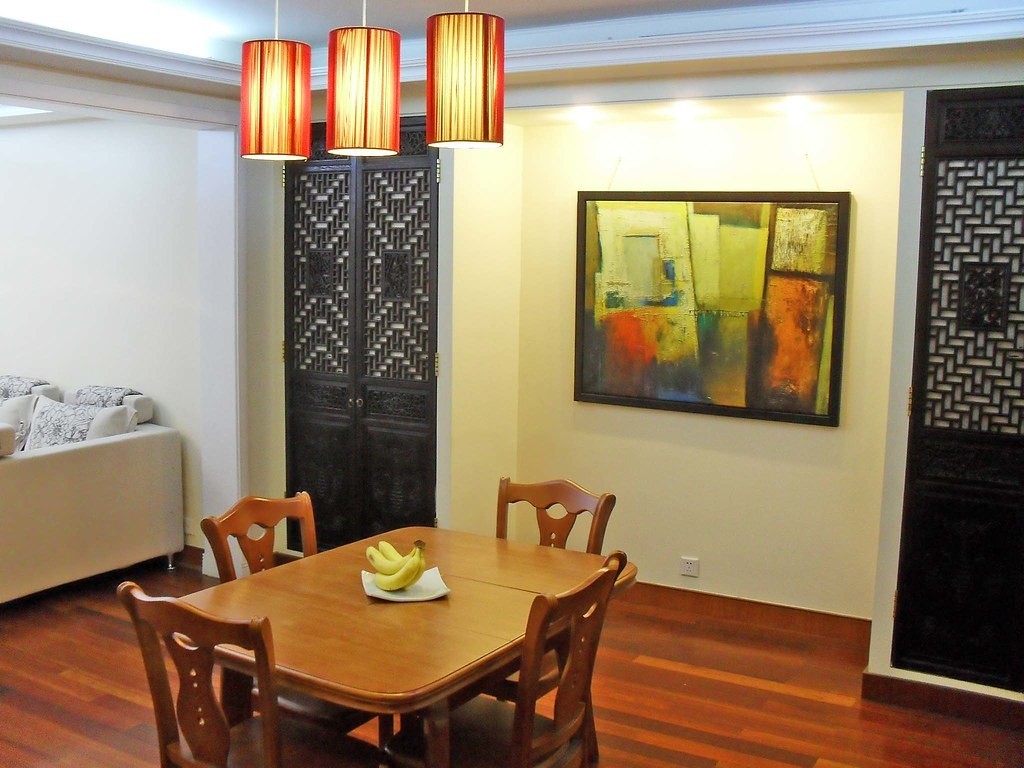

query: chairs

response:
[117,579,382,768]
[481,475,616,704]
[384,548,628,768]
[199,491,395,751]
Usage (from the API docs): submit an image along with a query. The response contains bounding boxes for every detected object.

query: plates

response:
[361,566,451,603]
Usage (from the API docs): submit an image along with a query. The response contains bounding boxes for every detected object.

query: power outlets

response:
[184,518,194,534]
[679,557,700,578]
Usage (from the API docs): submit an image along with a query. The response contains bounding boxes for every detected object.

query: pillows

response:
[0,394,39,452]
[22,394,128,452]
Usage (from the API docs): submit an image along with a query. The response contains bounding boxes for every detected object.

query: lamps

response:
[326,1,402,156]
[425,0,504,148]
[241,0,310,162]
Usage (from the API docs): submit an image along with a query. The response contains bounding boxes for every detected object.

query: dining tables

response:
[175,525,637,768]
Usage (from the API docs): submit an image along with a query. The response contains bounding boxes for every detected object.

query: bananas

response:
[366,538,426,590]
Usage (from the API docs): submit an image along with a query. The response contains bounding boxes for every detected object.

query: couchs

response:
[0,374,185,604]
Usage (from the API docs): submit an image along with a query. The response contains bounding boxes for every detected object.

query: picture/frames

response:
[573,190,851,428]
